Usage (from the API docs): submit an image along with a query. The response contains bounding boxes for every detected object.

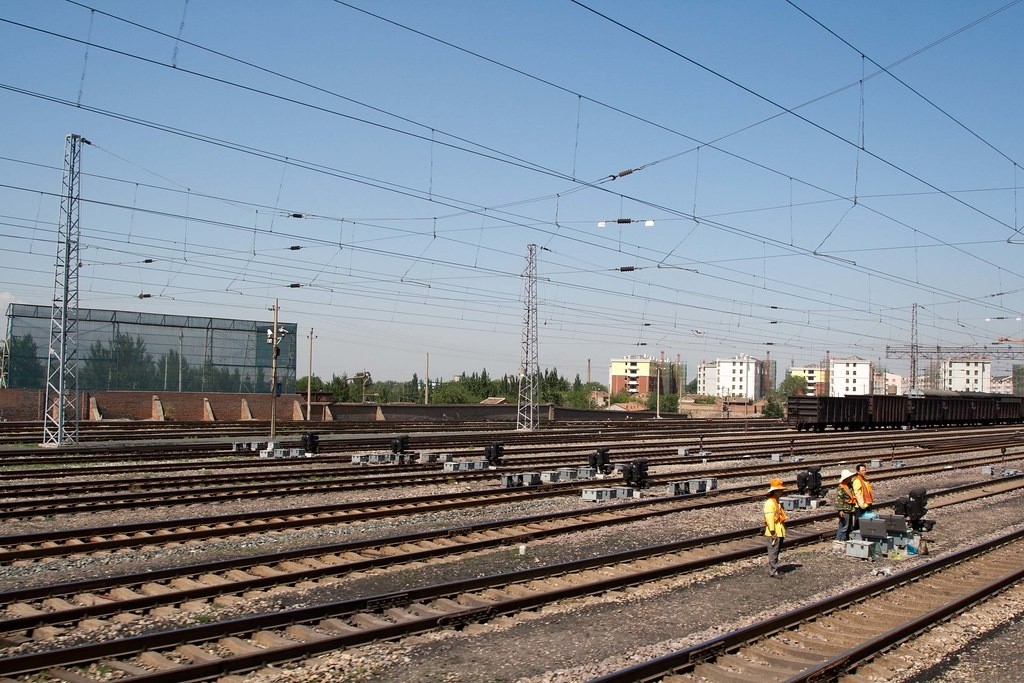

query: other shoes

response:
[772,570,783,579]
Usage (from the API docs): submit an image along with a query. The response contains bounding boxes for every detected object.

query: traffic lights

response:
[310,431,320,451]
[596,447,610,469]
[633,458,649,482]
[807,464,822,491]
[909,488,928,521]
[492,440,504,459]
[399,435,409,454]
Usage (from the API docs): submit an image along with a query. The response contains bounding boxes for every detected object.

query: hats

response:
[839,469,856,484]
[766,478,787,493]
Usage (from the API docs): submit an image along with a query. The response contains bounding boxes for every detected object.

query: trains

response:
[786,387,1024,433]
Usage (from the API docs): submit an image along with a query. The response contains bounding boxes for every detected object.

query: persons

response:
[835,469,856,541]
[852,465,873,529]
[763,478,787,579]
